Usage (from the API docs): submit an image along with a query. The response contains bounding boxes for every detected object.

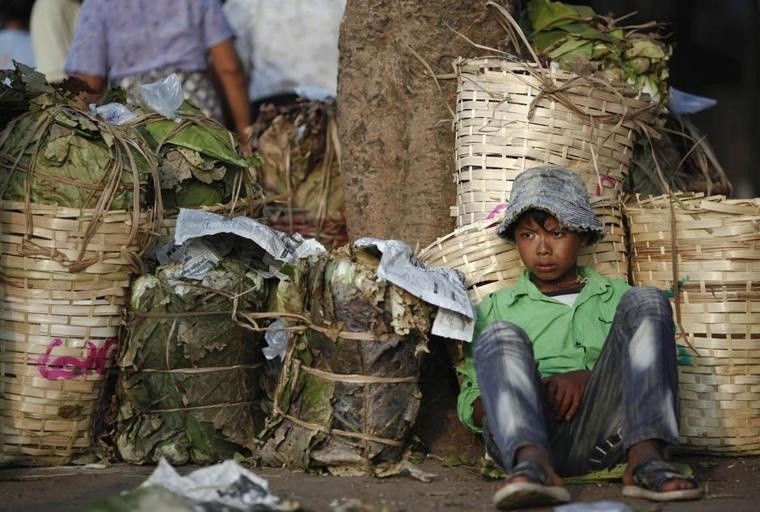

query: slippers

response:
[493,459,571,509]
[621,459,703,502]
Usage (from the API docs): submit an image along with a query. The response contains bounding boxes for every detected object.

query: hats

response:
[495,165,605,246]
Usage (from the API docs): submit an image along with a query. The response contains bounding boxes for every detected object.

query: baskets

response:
[417,57,760,456]
[415,54,760,456]
[0,199,140,466]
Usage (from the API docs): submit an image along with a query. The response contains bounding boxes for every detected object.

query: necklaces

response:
[553,268,588,288]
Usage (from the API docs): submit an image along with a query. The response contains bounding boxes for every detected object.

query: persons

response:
[0,0,83,85]
[222,0,345,121]
[456,166,704,509]
[65,0,253,153]
